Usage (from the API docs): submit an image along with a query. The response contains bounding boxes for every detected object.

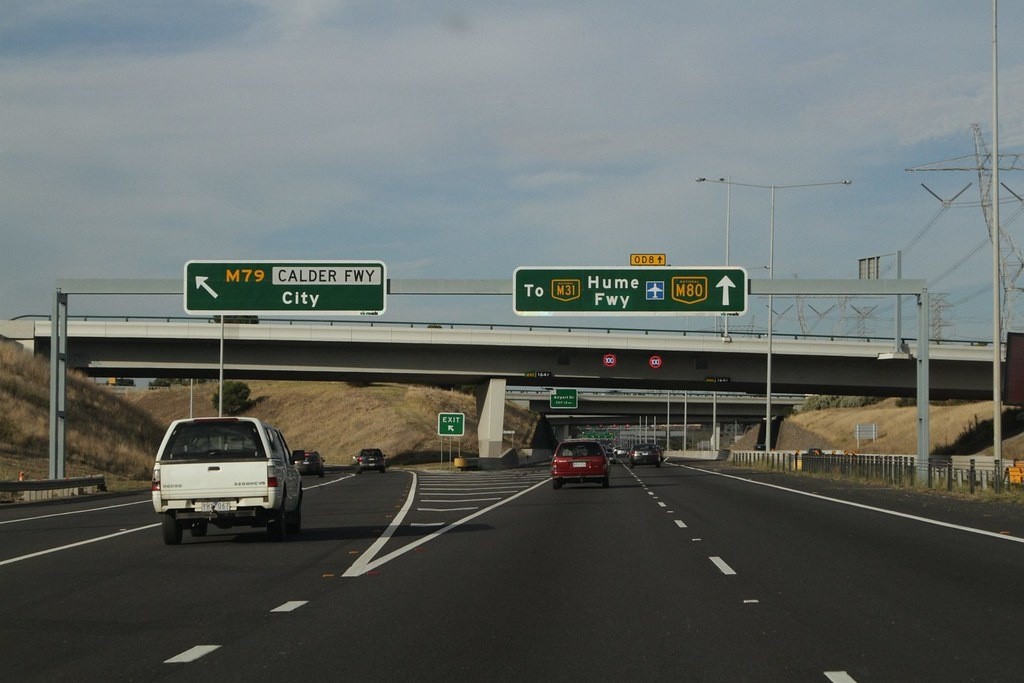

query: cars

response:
[549,440,613,489]
[293,451,327,477]
[626,445,662,469]
[602,442,629,464]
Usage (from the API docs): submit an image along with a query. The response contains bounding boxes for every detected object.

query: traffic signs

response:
[437,412,466,437]
[512,252,749,319]
[182,258,388,317]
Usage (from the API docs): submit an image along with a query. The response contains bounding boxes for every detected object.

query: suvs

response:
[353,448,387,474]
[149,414,305,545]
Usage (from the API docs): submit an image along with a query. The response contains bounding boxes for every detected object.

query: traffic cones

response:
[18,471,25,482]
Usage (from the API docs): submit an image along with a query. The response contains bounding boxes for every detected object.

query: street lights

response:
[719,175,730,337]
[694,176,853,454]
